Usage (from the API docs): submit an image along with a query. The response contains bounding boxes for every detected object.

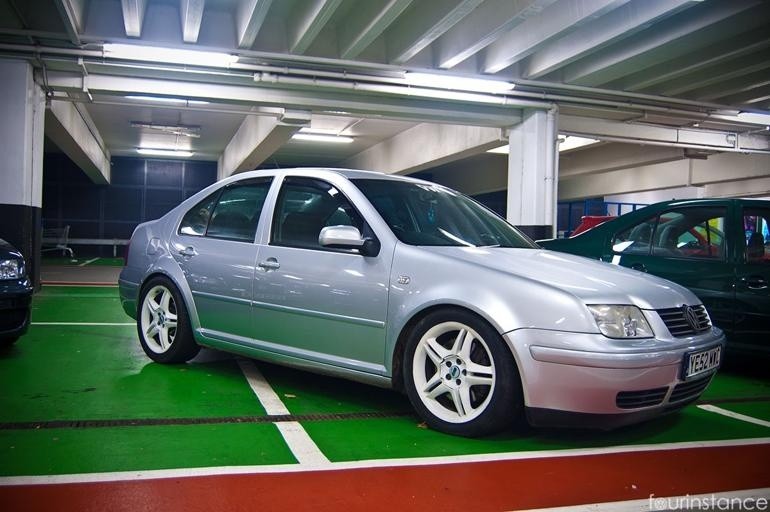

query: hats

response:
[117,166,726,440]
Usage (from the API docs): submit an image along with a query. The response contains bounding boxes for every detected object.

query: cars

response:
[117,166,726,440]
[532,197,770,375]
[566,215,768,261]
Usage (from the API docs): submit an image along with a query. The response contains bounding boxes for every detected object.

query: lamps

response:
[210,205,332,253]
[744,232,764,257]
[627,225,685,259]
